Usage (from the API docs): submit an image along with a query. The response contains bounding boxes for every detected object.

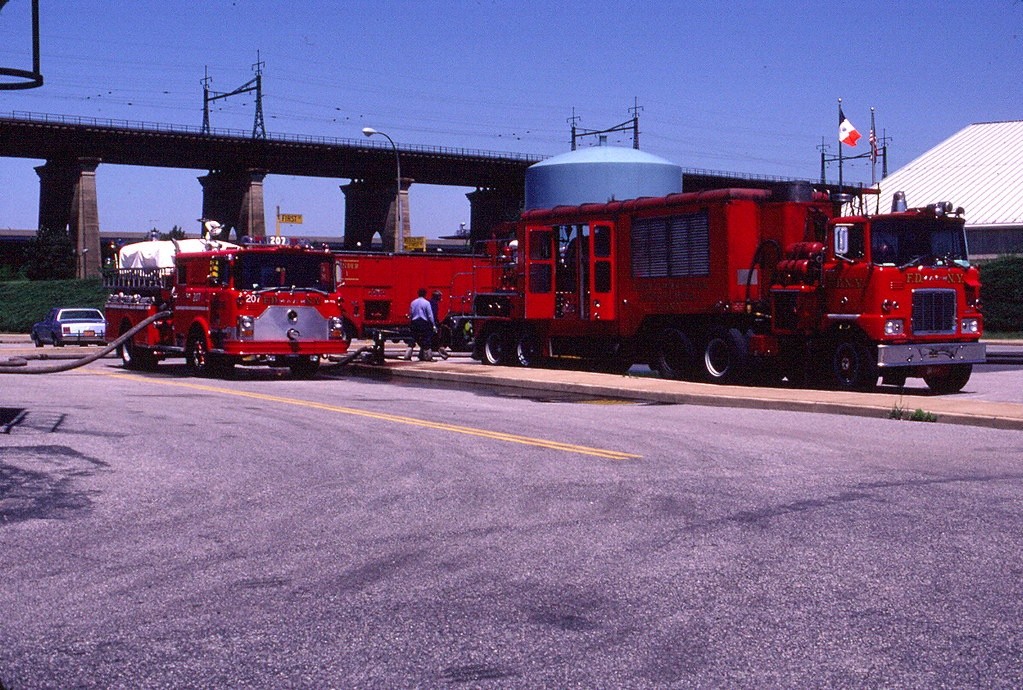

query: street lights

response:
[362,127,402,252]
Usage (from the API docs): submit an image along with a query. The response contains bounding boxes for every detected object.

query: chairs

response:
[564,236,589,267]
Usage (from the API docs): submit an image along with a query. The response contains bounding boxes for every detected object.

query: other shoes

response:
[444,355,452,360]
[419,357,426,361]
[404,358,412,361]
[426,357,437,362]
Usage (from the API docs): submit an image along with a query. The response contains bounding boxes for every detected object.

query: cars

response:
[30,307,109,347]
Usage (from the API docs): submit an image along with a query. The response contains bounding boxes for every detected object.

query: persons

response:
[404,288,449,364]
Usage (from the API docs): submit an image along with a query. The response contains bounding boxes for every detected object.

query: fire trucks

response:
[103,230,503,379]
[459,178,987,394]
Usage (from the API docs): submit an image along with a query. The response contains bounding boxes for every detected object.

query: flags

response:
[870,110,878,163]
[838,104,863,148]
